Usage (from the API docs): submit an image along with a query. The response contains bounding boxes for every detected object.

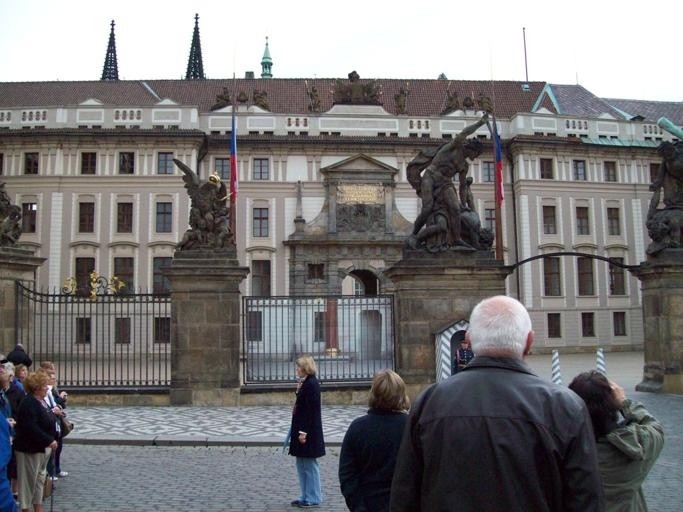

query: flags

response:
[494,122,505,209]
[230,104,238,204]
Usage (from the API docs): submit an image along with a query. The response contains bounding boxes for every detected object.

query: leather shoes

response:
[58,470,68,477]
[50,476,58,481]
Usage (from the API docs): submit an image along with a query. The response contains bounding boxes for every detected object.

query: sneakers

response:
[291,500,320,507]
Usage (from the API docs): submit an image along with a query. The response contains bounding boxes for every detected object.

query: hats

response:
[460,339,468,343]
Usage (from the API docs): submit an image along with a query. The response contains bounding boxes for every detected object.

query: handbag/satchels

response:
[61,416,73,436]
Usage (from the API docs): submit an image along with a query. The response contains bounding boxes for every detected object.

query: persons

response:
[394,85,410,116]
[172,157,237,253]
[0,343,75,512]
[454,338,473,374]
[644,138,683,256]
[473,91,492,113]
[440,90,460,116]
[287,355,326,509]
[337,366,411,511]
[235,91,249,104]
[346,71,367,105]
[0,181,23,244]
[253,88,271,113]
[403,112,495,251]
[332,79,349,104]
[462,96,475,108]
[210,86,232,110]
[389,293,606,512]
[306,86,321,113]
[568,371,665,512]
[362,81,383,106]
